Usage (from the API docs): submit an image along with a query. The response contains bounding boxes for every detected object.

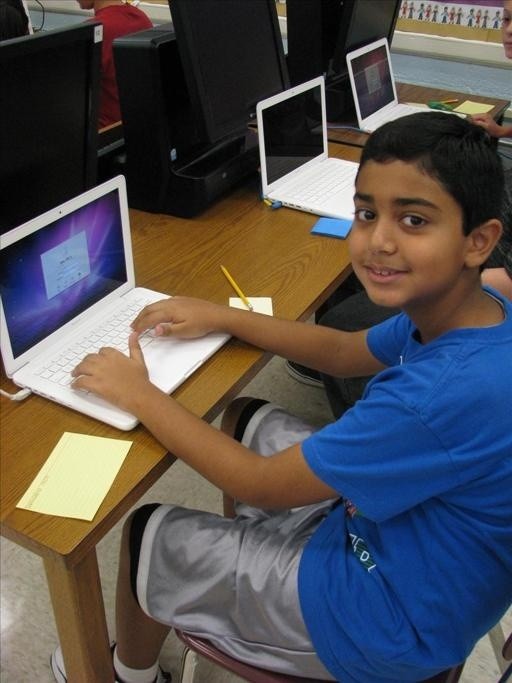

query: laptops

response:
[256,76,361,221]
[345,36,468,137]
[0,174,232,432]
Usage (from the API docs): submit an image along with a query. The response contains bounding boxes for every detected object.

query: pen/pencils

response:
[218,264,253,311]
[442,99,459,103]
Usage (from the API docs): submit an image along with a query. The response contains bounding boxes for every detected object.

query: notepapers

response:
[452,100,496,115]
[229,296,274,317]
[311,217,354,240]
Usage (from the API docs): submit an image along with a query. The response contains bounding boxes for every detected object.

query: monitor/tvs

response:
[168,1,292,148]
[338,0,403,53]
[0,17,105,233]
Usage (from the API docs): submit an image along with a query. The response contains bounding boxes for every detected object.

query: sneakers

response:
[50,640,171,683]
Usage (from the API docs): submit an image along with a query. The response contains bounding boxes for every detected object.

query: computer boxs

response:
[111,21,202,197]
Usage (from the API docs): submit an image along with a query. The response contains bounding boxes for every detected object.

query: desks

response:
[232,80,511,153]
[0,124,366,680]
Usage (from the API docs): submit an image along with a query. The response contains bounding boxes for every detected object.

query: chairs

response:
[171,624,511,683]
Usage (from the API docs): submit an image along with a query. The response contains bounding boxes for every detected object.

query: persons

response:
[50,111,511,682]
[76,0,155,132]
[464,112,510,137]
[500,0,511,61]
[284,145,511,421]
